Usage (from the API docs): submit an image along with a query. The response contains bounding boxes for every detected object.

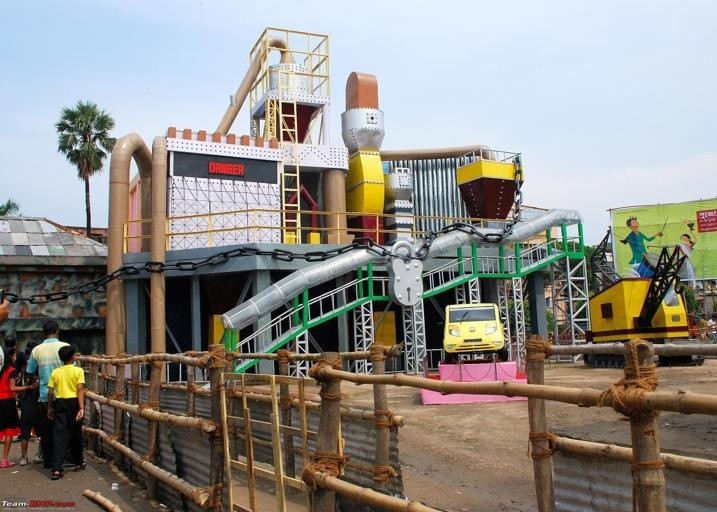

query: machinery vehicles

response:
[580,228,707,370]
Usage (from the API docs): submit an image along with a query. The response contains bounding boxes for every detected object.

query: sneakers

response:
[75,460,86,471]
[17,455,28,465]
[30,455,44,463]
[51,470,64,479]
[0,457,15,468]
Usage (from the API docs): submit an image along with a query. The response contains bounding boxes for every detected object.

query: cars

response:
[443,301,510,364]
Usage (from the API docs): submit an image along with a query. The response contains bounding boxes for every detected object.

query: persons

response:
[688,310,717,345]
[619,216,664,273]
[23,319,78,468]
[672,230,698,289]
[0,332,88,480]
